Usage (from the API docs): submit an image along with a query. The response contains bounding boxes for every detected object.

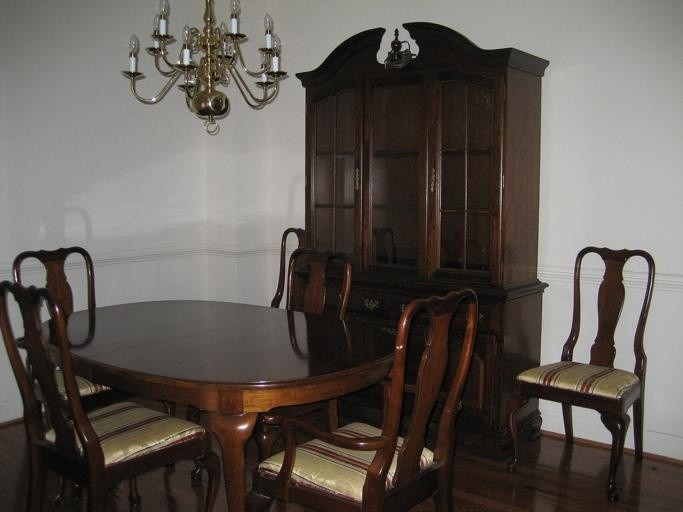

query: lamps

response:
[120,0,288,133]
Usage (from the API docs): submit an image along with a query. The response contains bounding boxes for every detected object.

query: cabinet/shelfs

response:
[285,19,551,462]
